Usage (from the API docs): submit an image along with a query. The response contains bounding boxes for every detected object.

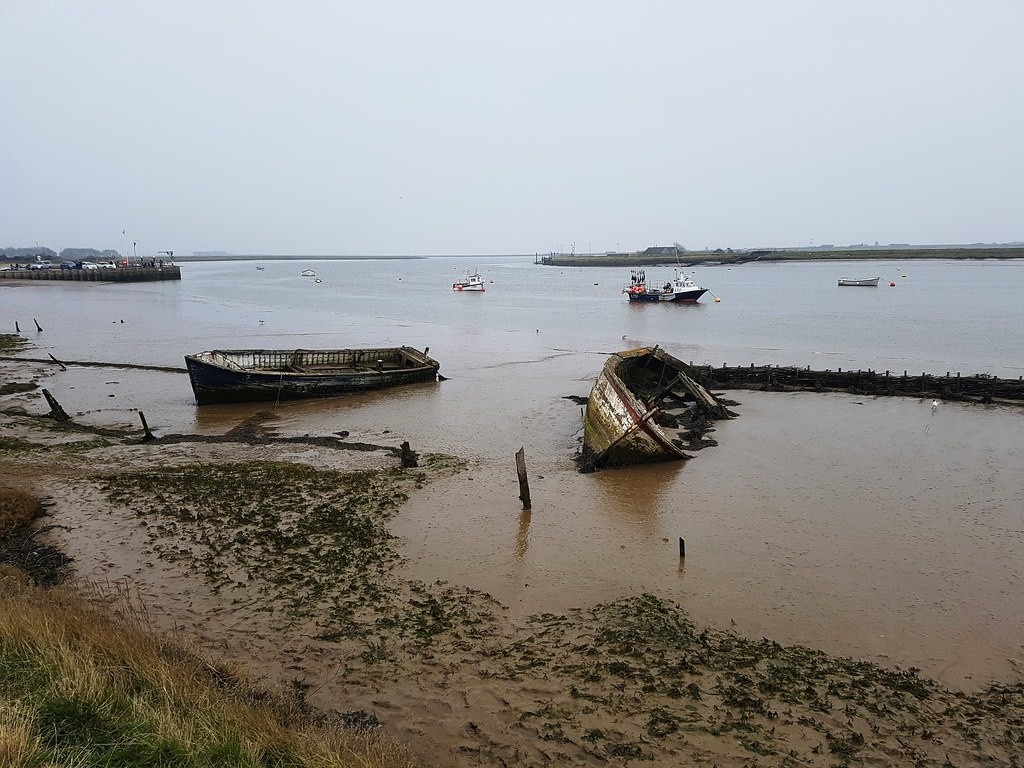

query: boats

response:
[582,343,704,467]
[184,343,440,406]
[838,276,880,286]
[452,267,485,291]
[620,269,710,302]
[301,268,316,276]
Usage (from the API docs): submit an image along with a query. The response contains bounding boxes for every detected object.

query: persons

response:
[150,260,155,268]
[15,263,19,271]
[10,263,14,271]
[159,259,163,270]
[932,399,940,410]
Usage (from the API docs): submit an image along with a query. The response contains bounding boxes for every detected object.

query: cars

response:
[59,261,116,270]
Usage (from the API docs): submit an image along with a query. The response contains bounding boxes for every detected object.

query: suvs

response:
[31,260,53,271]
